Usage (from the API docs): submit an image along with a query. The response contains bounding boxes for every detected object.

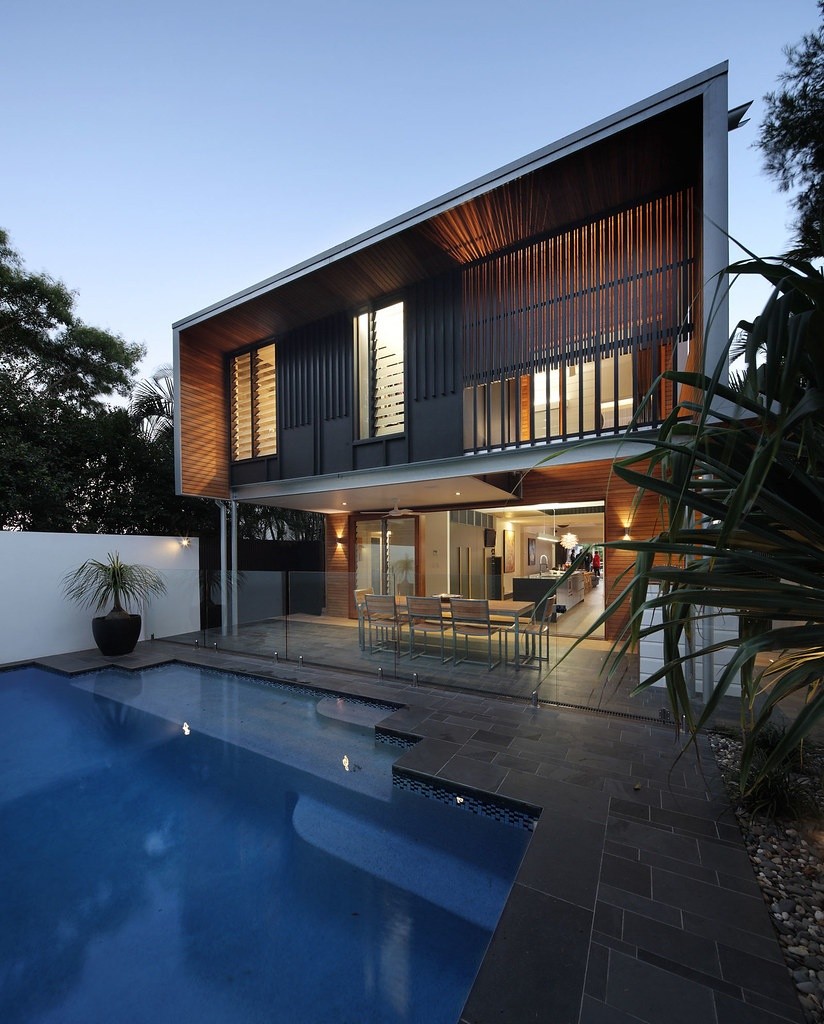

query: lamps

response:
[336,538,347,543]
[728,100,755,132]
[560,525,579,550]
[623,526,629,535]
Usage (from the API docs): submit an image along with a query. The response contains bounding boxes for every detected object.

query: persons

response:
[593,551,600,576]
[570,549,593,572]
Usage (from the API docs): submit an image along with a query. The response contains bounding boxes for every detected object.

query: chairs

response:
[504,595,556,671]
[449,597,501,672]
[353,587,416,659]
[406,596,458,665]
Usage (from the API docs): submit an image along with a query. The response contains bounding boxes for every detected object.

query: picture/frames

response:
[503,529,516,573]
[485,529,496,548]
[528,537,536,566]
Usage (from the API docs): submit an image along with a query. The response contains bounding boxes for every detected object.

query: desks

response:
[362,596,536,673]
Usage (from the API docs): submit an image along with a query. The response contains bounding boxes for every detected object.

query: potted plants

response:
[57,551,168,656]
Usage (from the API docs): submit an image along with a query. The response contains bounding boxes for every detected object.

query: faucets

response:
[539,554,549,577]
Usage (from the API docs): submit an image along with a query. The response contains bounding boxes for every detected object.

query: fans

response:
[359,497,422,518]
